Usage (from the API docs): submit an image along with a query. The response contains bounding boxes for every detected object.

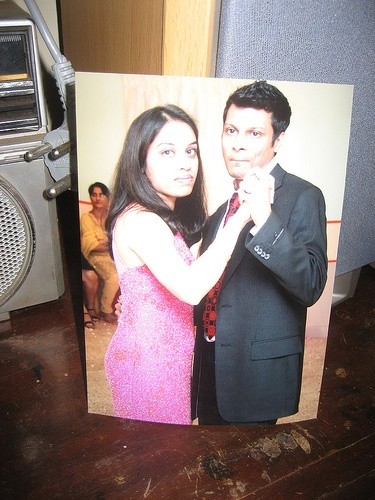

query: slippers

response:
[88,309,99,322]
[84,313,95,329]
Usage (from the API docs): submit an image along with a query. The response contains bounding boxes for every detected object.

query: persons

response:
[80,79,328,427]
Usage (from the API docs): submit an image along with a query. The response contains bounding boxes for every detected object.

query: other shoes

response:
[99,308,117,322]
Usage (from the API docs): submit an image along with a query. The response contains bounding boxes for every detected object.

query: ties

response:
[202,179,245,340]
